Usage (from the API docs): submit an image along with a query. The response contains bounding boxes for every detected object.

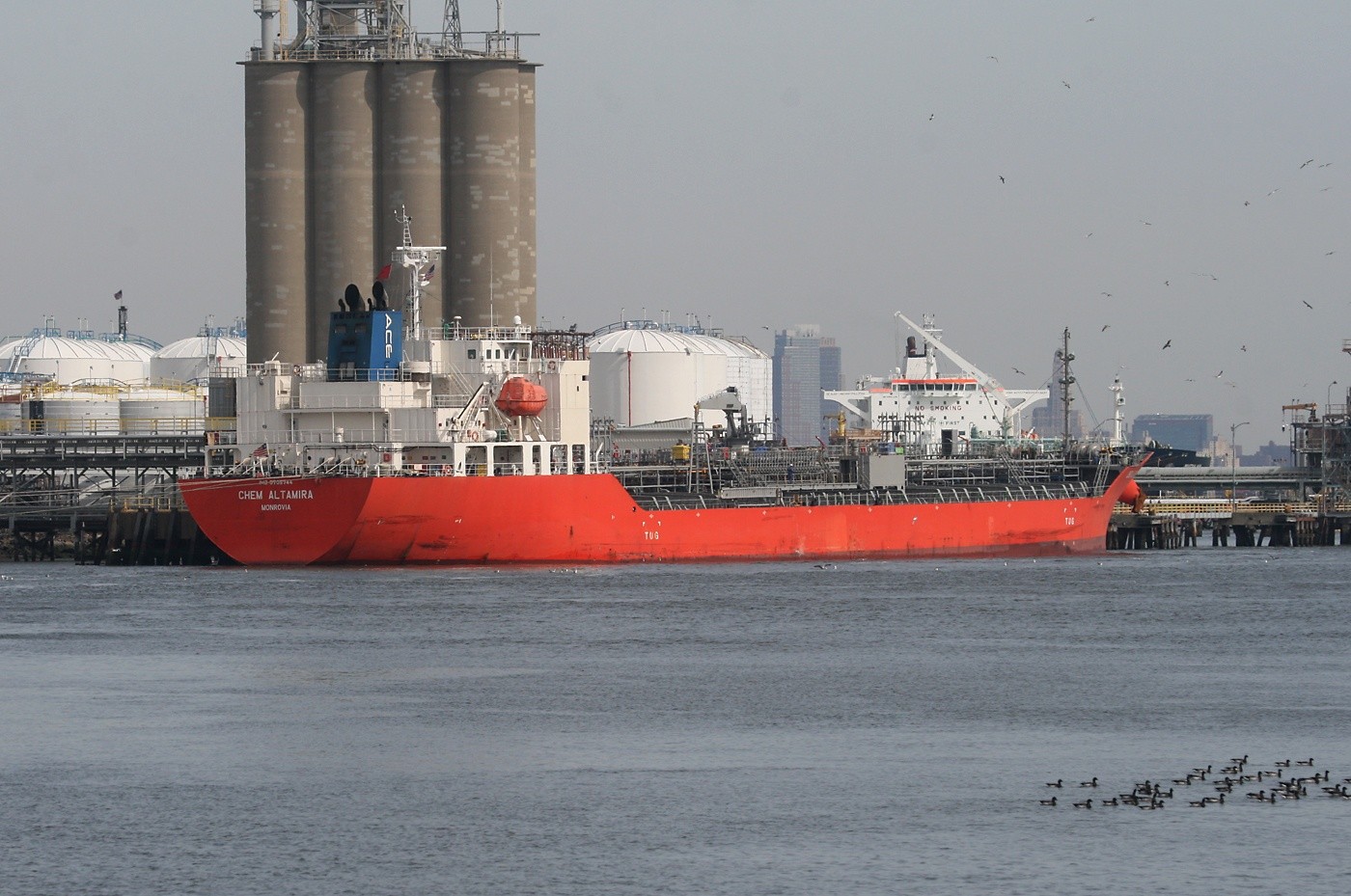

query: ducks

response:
[1039,755,1351,810]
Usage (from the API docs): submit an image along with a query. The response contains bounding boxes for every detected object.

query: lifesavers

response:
[472,432,479,442]
[384,454,390,462]
[292,365,301,374]
[445,466,452,476]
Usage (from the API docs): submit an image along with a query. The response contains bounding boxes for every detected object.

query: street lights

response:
[1231,422,1251,515]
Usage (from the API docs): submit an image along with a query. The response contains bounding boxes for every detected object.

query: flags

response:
[253,442,268,458]
[376,263,392,279]
[115,290,122,299]
[424,265,435,282]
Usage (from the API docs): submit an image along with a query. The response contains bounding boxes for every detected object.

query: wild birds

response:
[1300,159,1314,170]
[1319,162,1333,169]
[1011,366,1026,376]
[1062,80,1071,89]
[998,175,1005,184]
[1083,190,1351,388]
[986,56,999,61]
[929,114,934,121]
[1085,17,1096,22]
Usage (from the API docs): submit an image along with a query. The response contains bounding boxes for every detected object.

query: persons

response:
[336,455,341,464]
[612,442,620,464]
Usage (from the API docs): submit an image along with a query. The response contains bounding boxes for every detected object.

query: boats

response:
[494,376,550,417]
[175,198,1161,571]
